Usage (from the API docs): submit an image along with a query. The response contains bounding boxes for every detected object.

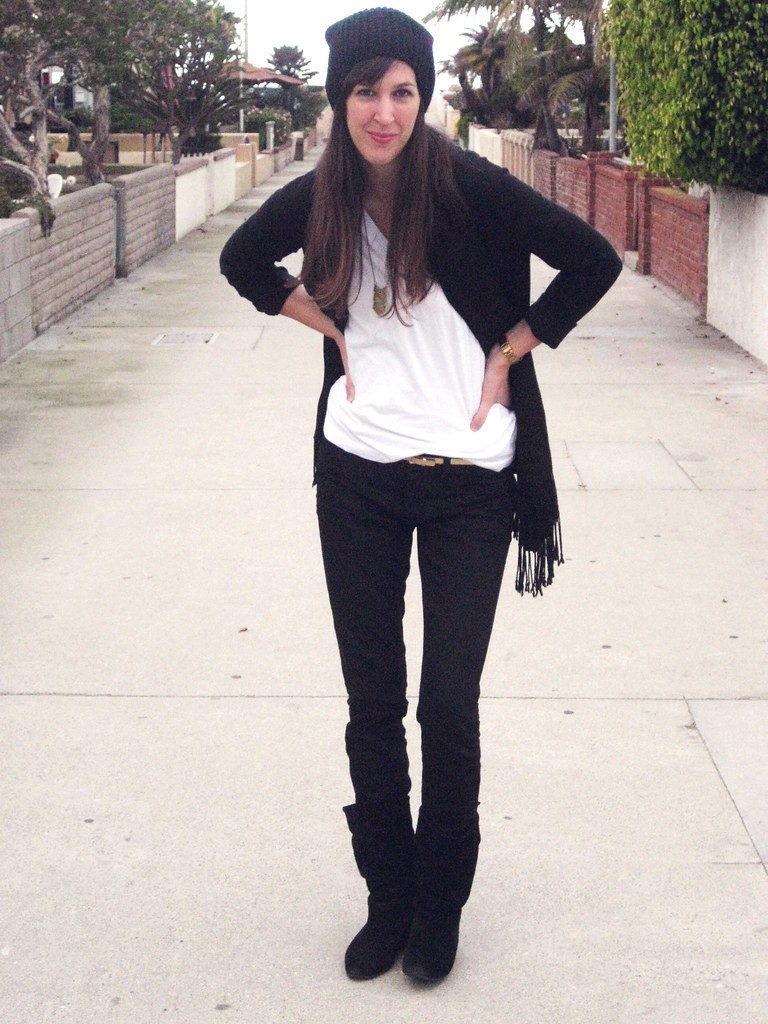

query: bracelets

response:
[501,334,520,364]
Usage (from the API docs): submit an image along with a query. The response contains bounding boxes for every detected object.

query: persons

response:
[219,7,621,980]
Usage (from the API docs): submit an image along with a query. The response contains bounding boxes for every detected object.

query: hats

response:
[325,6,435,113]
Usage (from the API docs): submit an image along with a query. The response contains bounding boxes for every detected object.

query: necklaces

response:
[360,200,394,315]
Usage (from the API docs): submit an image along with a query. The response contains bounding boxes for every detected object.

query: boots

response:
[343,797,415,981]
[401,805,481,981]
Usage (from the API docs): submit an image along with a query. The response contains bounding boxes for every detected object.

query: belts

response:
[404,454,475,466]
